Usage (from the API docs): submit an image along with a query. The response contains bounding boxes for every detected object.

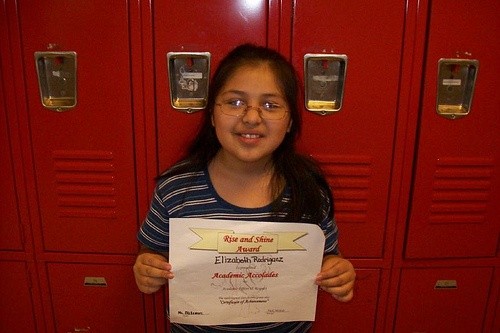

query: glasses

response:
[213,96,291,120]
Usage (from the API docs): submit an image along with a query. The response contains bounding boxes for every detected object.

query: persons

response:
[134,42,356,333]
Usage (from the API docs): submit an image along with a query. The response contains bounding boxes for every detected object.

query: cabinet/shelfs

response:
[0,0,500,333]
